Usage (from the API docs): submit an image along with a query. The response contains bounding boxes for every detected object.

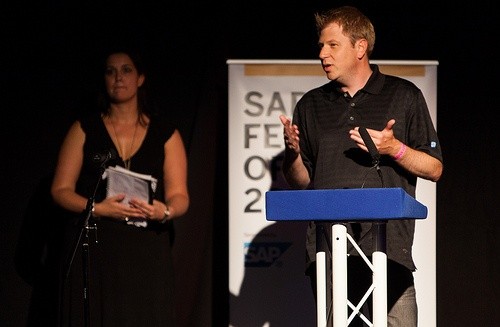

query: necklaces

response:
[110,116,138,170]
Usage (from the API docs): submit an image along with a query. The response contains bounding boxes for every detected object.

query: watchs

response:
[160,205,170,224]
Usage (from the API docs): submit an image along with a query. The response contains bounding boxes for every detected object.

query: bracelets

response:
[91,200,96,217]
[391,142,407,160]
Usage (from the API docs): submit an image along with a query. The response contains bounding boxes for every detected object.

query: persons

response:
[50,51,189,327]
[279,6,444,327]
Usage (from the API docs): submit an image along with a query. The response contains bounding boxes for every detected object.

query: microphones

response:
[93,146,116,163]
[358,127,381,189]
